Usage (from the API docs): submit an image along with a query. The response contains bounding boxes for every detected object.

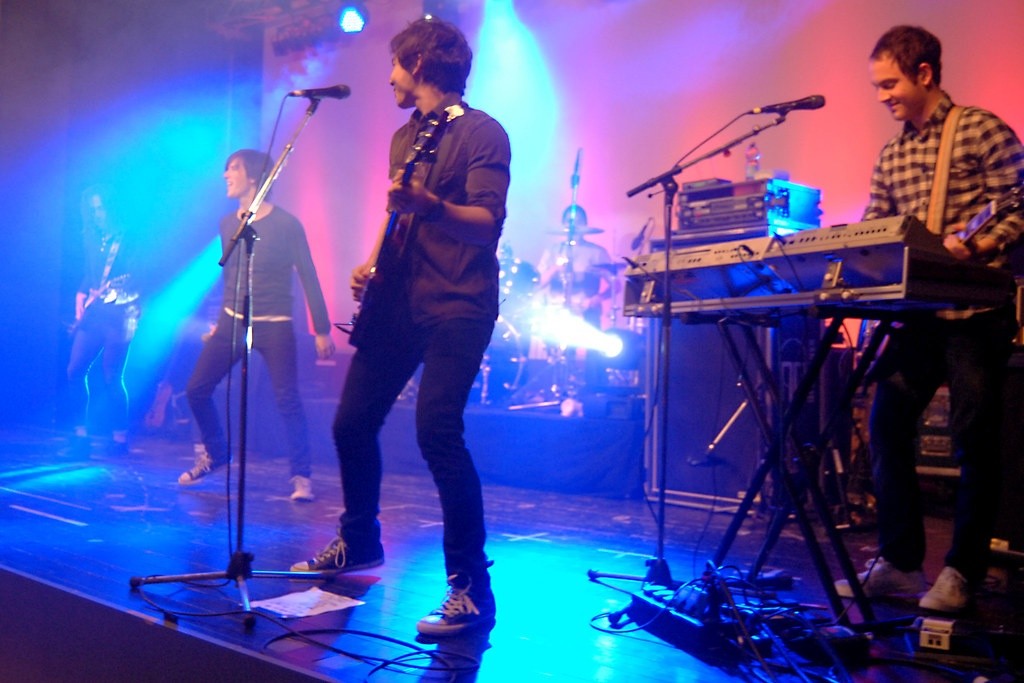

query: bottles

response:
[745,142,760,180]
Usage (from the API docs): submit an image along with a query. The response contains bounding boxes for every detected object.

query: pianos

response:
[620,212,980,636]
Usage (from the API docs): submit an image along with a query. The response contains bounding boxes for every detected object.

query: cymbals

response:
[547,225,604,235]
[592,262,647,270]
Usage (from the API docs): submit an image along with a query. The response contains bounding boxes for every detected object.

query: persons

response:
[179,148,336,503]
[831,25,1024,612]
[288,16,512,627]
[58,184,141,460]
[538,205,622,360]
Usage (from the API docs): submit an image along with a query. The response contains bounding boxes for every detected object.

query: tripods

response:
[509,181,586,406]
[588,112,787,624]
[130,99,336,629]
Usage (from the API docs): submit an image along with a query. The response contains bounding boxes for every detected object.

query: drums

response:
[465,311,532,408]
[497,258,542,308]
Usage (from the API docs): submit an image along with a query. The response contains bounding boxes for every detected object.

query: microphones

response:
[748,95,825,115]
[288,84,350,100]
[631,223,649,251]
[571,151,580,186]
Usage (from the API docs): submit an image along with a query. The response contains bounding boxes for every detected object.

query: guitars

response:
[68,272,133,337]
[856,183,1024,382]
[348,102,466,351]
[144,340,185,429]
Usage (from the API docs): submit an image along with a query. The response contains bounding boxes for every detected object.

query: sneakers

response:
[290,536,384,582]
[914,565,973,620]
[833,556,919,599]
[178,454,233,485]
[417,585,496,636]
[288,475,315,502]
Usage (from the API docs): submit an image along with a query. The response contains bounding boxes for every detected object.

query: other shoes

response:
[57,436,95,456]
[88,440,130,460]
